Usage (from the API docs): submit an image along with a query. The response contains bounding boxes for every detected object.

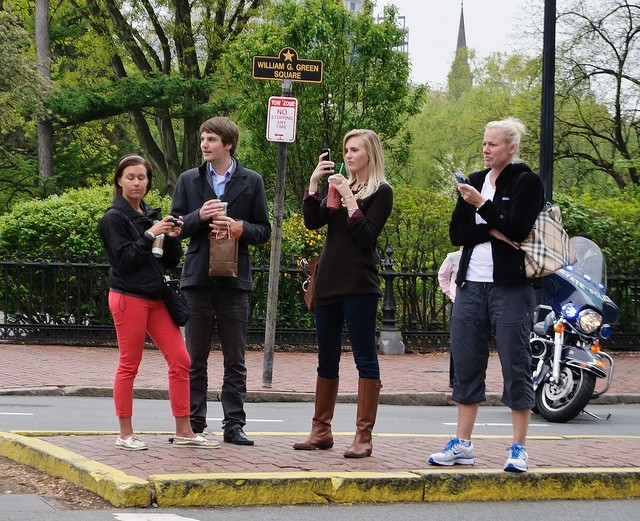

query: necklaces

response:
[342,181,367,207]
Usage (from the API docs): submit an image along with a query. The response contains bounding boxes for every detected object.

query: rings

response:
[460,193,464,197]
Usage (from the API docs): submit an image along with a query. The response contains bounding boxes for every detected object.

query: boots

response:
[293,374,338,450]
[343,375,384,458]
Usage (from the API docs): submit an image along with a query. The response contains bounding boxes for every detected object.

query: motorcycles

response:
[532,236,619,422]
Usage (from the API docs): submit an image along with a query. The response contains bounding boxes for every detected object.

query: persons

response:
[170,116,271,445]
[430,116,545,471]
[293,126,394,458]
[98,154,221,450]
[437,243,464,389]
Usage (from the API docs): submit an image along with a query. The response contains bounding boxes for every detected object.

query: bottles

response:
[152,232,164,258]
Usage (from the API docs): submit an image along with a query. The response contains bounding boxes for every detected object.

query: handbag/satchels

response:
[511,171,579,281]
[301,254,324,312]
[206,223,241,280]
[162,264,194,328]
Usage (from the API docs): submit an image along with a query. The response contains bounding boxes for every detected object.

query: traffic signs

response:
[251,45,324,84]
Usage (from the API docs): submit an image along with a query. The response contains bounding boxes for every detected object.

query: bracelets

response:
[345,206,360,211]
[340,194,355,200]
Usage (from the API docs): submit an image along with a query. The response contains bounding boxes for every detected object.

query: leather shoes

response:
[223,420,255,446]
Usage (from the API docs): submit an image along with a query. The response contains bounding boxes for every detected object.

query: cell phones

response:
[454,171,465,183]
[322,148,331,170]
[168,218,182,228]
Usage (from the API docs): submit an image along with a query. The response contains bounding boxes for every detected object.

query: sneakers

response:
[504,443,529,474]
[428,436,475,467]
[115,433,149,452]
[172,430,220,450]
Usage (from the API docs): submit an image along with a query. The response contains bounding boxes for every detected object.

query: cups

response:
[211,202,228,240]
[326,183,341,210]
